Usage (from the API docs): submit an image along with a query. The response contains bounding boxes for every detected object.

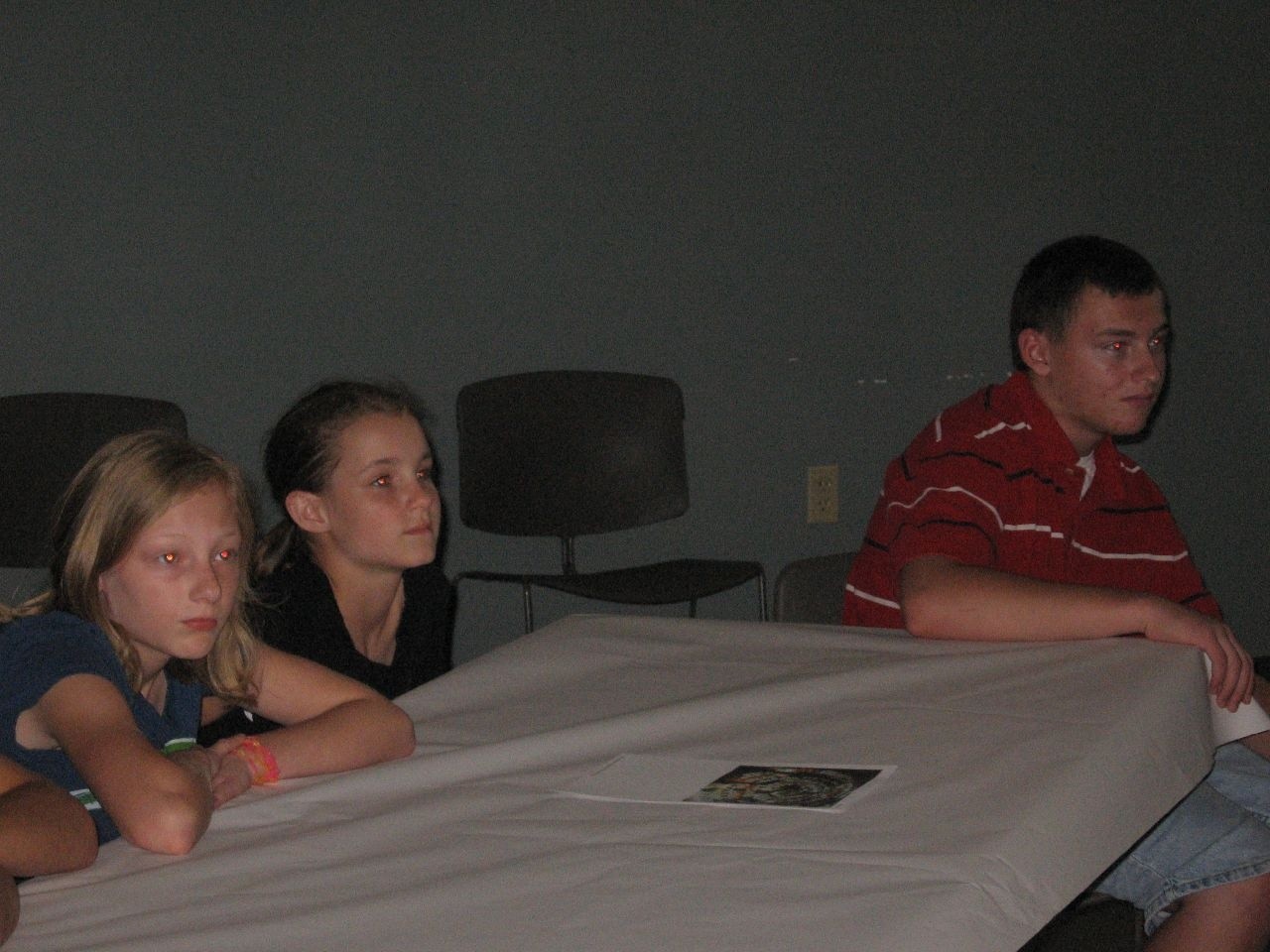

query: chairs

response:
[0,392,192,580]
[779,553,1143,952]
[455,371,767,630]
[0,614,1223,952]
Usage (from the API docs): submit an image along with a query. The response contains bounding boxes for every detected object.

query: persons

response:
[199,380,461,747]
[840,234,1270,952]
[0,428,417,947]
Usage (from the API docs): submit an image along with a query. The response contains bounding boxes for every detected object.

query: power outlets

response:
[808,462,840,525]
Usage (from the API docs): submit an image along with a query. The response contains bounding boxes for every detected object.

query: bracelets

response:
[229,736,282,786]
